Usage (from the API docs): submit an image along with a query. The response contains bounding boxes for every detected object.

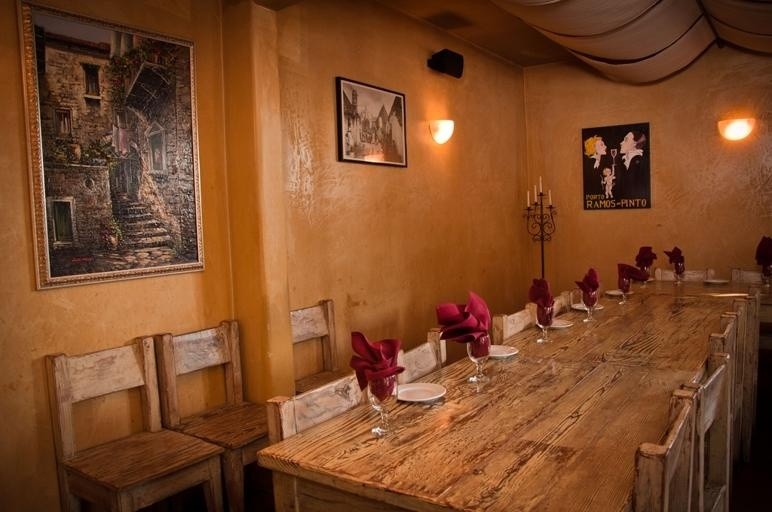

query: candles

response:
[526,175,552,208]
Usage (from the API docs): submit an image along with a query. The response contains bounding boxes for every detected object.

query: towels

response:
[634,245,685,274]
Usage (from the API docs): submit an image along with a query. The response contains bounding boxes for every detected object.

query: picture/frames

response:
[335,76,408,169]
[17,1,206,293]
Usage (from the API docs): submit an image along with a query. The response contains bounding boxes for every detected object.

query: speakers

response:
[427,48,464,78]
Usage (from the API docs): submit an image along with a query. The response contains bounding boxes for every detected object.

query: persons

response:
[618,130,650,207]
[584,134,610,209]
[600,162,617,200]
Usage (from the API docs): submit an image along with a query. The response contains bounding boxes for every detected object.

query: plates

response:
[605,289,635,296]
[645,277,656,282]
[538,317,573,329]
[572,303,605,312]
[397,382,445,402]
[702,279,729,284]
[489,344,518,359]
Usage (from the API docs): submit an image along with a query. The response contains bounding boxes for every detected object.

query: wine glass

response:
[611,148,618,166]
[618,277,630,304]
[640,265,650,288]
[535,306,553,344]
[583,290,599,323]
[674,262,686,284]
[762,266,772,288]
[467,333,492,381]
[368,374,403,434]
